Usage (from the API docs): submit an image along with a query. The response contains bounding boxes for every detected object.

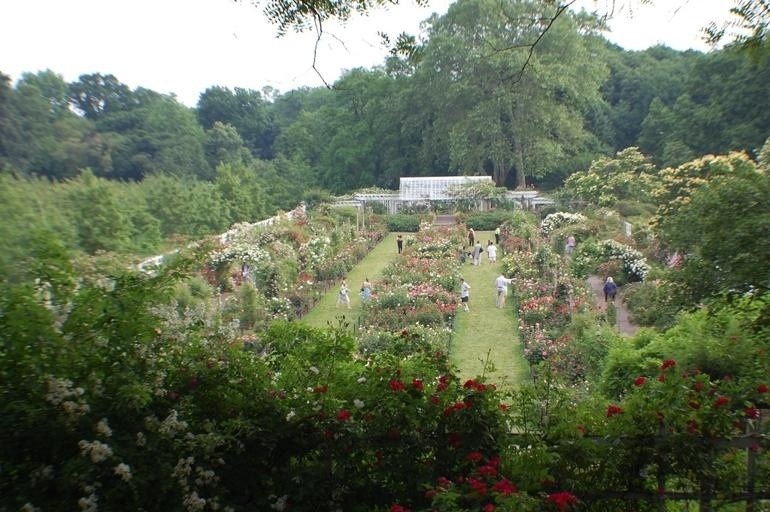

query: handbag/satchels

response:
[479,248,483,253]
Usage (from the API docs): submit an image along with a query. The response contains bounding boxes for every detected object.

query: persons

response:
[495,273,517,308]
[396,235,403,254]
[459,223,503,266]
[361,276,372,311]
[337,275,352,313]
[603,277,616,307]
[566,235,576,252]
[460,277,472,312]
